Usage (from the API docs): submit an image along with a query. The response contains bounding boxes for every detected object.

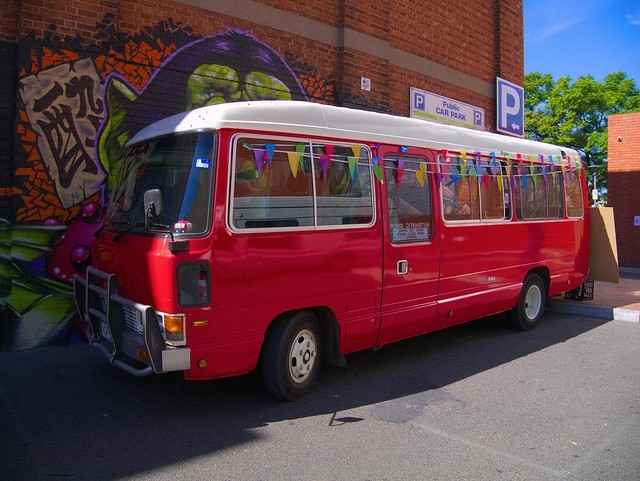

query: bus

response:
[74,100,590,401]
